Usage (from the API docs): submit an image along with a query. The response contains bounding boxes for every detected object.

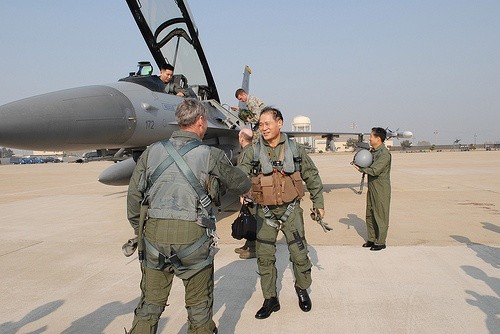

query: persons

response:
[350,127,391,251]
[235,128,258,259]
[231,89,267,130]
[125,98,252,334]
[158,65,189,97]
[234,107,325,318]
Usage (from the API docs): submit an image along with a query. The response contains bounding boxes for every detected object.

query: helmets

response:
[353,149,373,168]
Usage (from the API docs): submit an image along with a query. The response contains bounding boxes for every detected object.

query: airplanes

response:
[0,0,413,195]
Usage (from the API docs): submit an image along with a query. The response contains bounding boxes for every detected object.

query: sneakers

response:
[239,250,256,259]
[235,246,249,254]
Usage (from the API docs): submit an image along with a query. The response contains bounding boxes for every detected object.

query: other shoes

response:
[213,327,217,334]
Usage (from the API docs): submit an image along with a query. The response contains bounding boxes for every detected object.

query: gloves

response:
[122,236,139,257]
[309,208,333,232]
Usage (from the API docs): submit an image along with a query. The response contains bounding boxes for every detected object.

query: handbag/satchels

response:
[232,201,256,241]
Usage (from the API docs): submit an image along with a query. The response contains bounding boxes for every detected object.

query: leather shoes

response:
[362,241,374,247]
[295,280,311,312]
[370,244,386,250]
[255,296,280,319]
